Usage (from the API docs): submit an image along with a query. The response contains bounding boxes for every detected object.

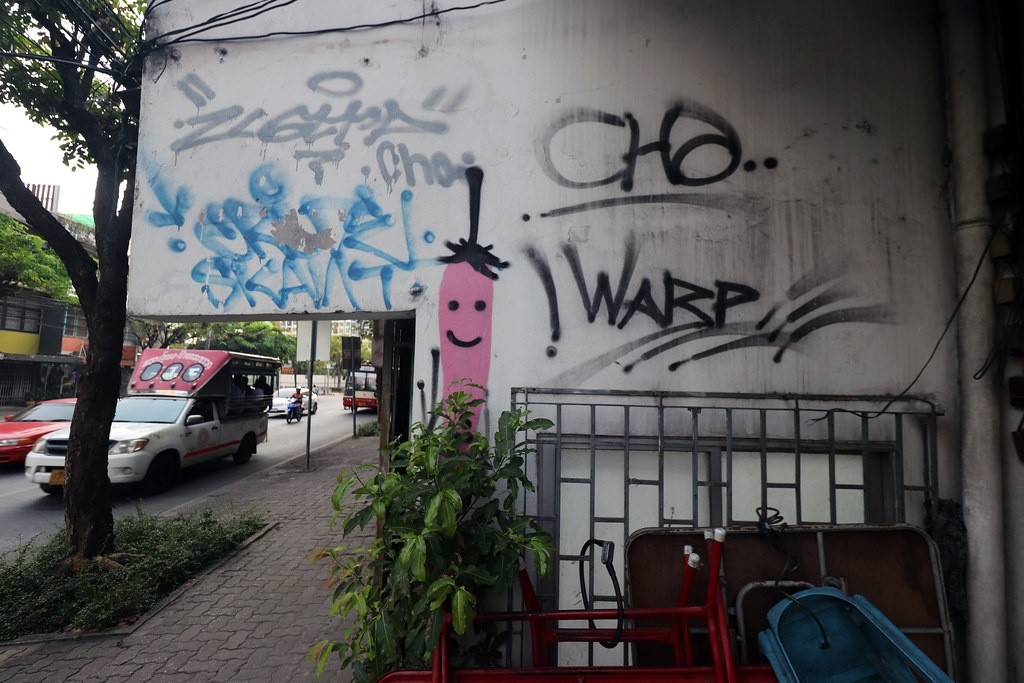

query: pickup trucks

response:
[23,345,284,498]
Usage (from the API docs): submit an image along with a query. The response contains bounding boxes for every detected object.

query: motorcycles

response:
[284,397,305,425]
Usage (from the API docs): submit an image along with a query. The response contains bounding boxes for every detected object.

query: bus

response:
[342,366,379,413]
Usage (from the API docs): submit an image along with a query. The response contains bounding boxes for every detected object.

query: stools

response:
[759,586,957,683]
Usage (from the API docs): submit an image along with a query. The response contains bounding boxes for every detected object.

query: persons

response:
[290,387,303,419]
[344,383,353,396]
[312,384,319,400]
[232,375,271,404]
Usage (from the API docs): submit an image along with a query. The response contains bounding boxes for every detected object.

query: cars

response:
[265,387,319,418]
[0,396,78,472]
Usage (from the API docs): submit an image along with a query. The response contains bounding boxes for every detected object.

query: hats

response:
[296,387,301,390]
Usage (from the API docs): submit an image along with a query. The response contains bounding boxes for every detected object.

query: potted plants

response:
[24,392,35,407]
